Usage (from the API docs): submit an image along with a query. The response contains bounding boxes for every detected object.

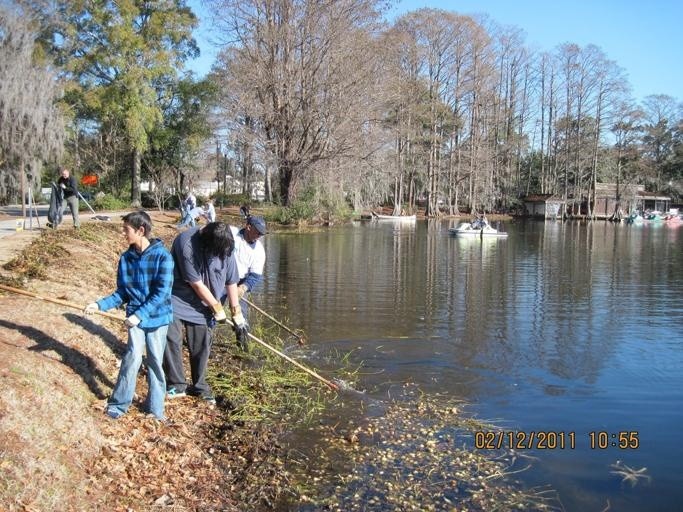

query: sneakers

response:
[159,418,172,427]
[166,387,186,398]
[46,223,57,230]
[193,391,213,400]
[107,407,119,418]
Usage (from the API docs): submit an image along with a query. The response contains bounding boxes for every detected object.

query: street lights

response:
[221,148,229,203]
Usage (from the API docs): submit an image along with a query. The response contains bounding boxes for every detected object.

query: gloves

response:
[230,304,250,351]
[207,302,226,324]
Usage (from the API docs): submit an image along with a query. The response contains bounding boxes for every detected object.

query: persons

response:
[84,211,175,423]
[56,169,79,228]
[168,222,248,404]
[207,214,265,355]
[399,209,405,214]
[168,188,215,228]
[476,215,485,227]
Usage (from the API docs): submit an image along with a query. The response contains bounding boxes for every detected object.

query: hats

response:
[248,216,266,235]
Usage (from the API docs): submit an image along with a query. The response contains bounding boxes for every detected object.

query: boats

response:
[626,209,681,222]
[377,219,415,223]
[455,233,499,258]
[630,222,682,230]
[447,213,508,237]
[374,213,415,219]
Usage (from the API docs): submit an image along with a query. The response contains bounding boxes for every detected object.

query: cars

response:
[78,185,100,202]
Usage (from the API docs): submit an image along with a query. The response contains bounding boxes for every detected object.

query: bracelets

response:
[239,284,247,292]
[231,305,241,314]
